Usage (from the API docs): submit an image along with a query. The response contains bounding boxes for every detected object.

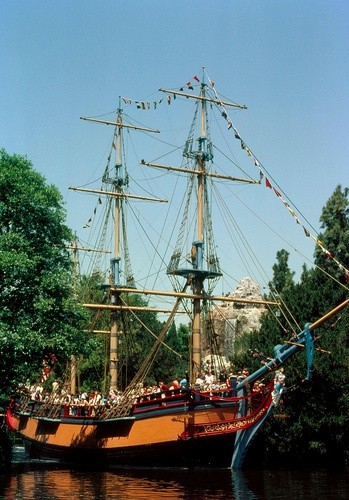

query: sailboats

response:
[5,65,349,449]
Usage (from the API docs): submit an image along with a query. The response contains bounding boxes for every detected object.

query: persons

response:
[17,366,265,420]
[270,367,286,406]
